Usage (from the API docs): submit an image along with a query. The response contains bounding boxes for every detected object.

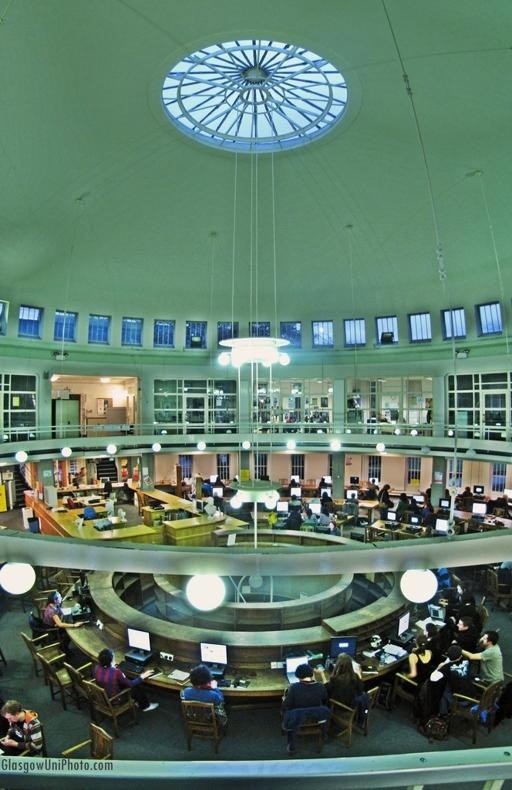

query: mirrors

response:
[95,397,115,417]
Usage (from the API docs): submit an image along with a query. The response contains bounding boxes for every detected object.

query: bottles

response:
[380,653,385,665]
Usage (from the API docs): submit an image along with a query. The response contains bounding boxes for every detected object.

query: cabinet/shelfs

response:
[52,399,81,438]
[106,406,128,435]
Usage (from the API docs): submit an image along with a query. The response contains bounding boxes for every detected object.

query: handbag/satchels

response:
[425,716,449,742]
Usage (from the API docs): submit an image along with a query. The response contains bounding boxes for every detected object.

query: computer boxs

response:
[386,629,413,646]
[72,606,92,622]
[198,662,225,678]
[124,648,154,664]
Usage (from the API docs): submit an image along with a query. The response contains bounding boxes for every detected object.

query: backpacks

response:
[375,681,396,712]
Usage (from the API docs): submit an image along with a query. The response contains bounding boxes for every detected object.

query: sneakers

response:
[141,701,160,713]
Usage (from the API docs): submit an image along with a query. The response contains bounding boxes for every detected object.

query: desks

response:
[85,417,109,437]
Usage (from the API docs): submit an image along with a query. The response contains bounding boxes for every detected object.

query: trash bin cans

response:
[28,518,39,533]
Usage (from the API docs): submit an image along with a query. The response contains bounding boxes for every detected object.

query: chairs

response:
[0,473,512,762]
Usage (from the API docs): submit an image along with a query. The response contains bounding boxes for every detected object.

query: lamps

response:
[189,333,203,348]
[213,73,294,371]
[380,331,396,345]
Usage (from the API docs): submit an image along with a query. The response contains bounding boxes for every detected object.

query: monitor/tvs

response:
[127,610,410,669]
[196,474,486,533]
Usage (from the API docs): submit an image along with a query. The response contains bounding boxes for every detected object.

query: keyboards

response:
[215,678,231,688]
[117,661,143,674]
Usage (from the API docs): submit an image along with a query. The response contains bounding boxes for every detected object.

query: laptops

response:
[286,655,309,684]
